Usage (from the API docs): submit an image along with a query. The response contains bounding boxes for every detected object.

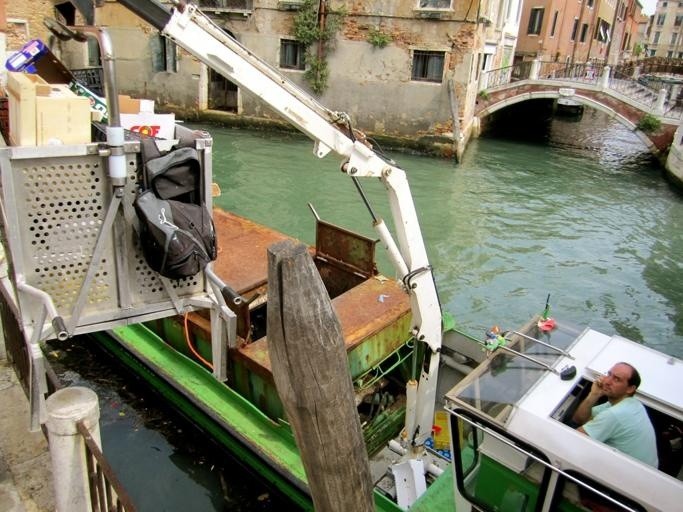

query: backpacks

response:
[133,137,217,279]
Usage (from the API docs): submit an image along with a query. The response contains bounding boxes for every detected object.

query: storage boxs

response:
[30,83,91,147]
[117,93,139,114]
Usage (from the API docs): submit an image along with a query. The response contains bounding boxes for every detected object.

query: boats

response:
[555,96,583,116]
[43,0,682,511]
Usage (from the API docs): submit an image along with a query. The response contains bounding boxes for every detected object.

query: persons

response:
[582,57,594,82]
[572,360,658,472]
